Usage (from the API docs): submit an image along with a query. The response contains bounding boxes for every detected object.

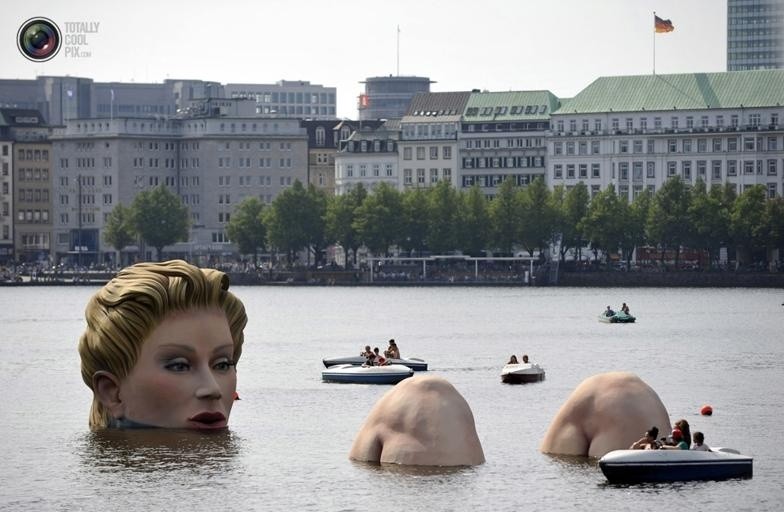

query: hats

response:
[668,429,682,437]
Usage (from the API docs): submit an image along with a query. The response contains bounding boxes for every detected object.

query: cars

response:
[0,252,784,274]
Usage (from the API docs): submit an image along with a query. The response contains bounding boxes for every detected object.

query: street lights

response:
[73,173,82,269]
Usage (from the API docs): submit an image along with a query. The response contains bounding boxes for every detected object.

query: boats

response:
[321,356,428,372]
[599,309,636,324]
[321,362,415,384]
[501,362,544,382]
[598,446,753,482]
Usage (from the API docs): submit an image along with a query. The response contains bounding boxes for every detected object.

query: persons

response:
[360,338,400,368]
[78,256,673,468]
[507,354,519,364]
[523,354,528,363]
[605,305,614,316]
[629,419,714,452]
[621,302,630,315]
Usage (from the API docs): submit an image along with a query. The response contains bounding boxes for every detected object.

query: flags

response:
[655,15,674,34]
